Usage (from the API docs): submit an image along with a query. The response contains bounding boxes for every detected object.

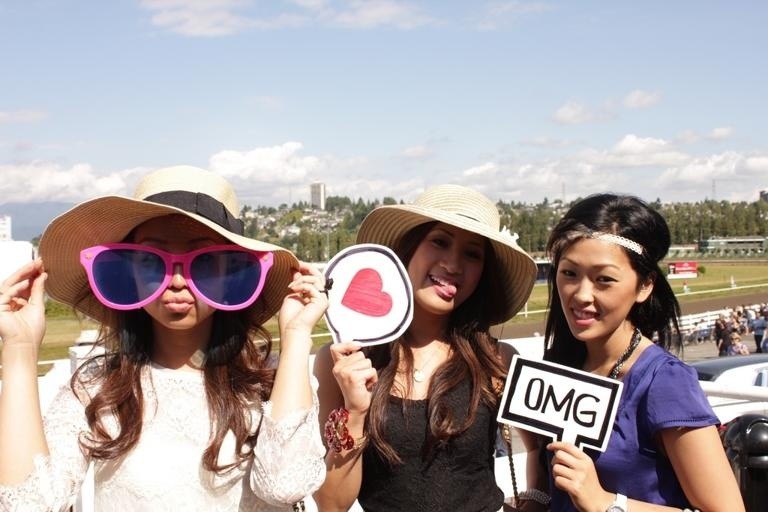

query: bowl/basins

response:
[606,328,641,381]
[412,354,431,381]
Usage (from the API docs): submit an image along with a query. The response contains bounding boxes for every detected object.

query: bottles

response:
[34,164,301,330]
[728,332,743,342]
[355,182,538,325]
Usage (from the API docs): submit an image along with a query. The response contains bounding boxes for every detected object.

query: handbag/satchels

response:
[518,487,551,507]
[324,407,366,455]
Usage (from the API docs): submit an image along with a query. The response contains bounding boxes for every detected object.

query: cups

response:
[75,242,274,311]
[731,337,739,340]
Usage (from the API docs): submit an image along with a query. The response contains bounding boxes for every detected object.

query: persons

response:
[311,185,540,512]
[1,166,330,512]
[659,300,768,358]
[533,192,750,512]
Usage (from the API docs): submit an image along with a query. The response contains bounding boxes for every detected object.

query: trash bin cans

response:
[606,492,628,512]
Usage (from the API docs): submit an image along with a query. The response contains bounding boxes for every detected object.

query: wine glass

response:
[319,289,327,293]
